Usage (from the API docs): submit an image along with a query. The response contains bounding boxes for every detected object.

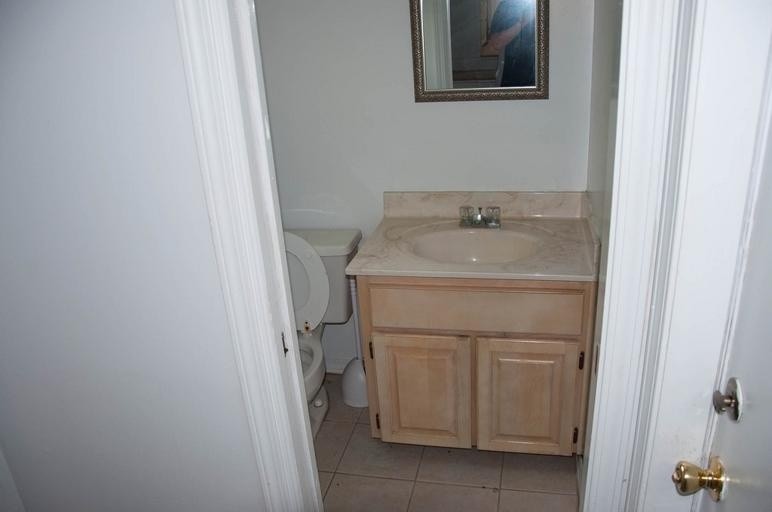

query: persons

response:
[488,1,534,86]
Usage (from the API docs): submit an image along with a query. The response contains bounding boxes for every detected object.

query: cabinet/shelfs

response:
[354,275,596,457]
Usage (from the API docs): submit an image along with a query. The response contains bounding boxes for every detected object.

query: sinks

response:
[406,224,545,266]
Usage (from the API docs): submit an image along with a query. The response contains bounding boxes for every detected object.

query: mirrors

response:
[410,0,552,103]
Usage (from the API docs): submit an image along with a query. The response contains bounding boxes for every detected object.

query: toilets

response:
[282,227,362,441]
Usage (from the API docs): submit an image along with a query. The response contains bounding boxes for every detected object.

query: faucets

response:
[458,204,501,228]
